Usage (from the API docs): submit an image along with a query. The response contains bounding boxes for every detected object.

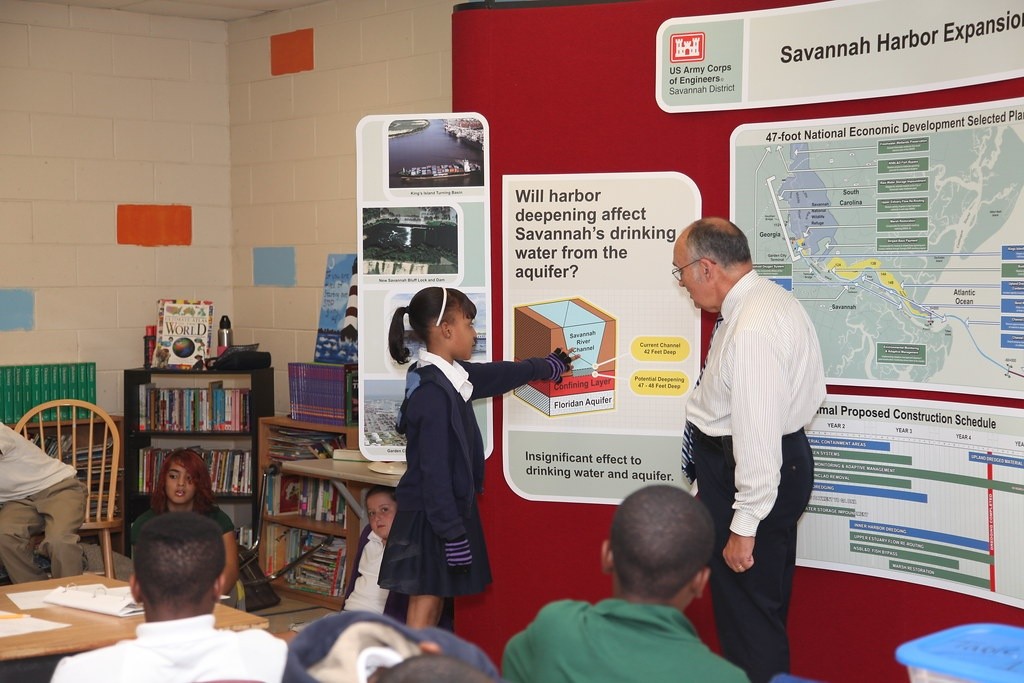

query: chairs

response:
[14,398,121,582]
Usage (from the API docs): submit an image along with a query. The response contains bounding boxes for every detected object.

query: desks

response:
[0,571,270,683]
[283,458,410,537]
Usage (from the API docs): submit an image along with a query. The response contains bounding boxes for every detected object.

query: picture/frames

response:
[156,298,214,372]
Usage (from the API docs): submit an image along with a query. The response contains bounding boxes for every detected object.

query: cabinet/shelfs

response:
[6,414,124,559]
[258,415,375,612]
[123,363,274,561]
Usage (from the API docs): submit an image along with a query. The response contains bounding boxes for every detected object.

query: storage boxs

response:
[896,621,1024,683]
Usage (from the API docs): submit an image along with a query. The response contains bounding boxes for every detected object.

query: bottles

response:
[218,315,234,355]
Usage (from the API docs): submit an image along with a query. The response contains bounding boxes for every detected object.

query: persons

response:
[0,421,89,585]
[282,610,503,683]
[502,484,750,683]
[272,484,409,644]
[127,450,240,607]
[48,512,287,683]
[376,287,582,630]
[673,215,825,683]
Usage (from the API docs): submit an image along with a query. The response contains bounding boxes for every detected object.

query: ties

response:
[681,313,724,485]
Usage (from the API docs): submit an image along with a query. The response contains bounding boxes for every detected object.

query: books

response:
[263,475,347,530]
[19,429,123,516]
[267,428,344,463]
[287,362,359,427]
[264,524,348,597]
[139,447,253,494]
[0,362,97,424]
[139,380,250,432]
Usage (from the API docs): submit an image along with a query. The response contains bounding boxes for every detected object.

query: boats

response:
[393,157,481,181]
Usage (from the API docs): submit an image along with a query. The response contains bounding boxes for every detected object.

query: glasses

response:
[672,257,717,281]
